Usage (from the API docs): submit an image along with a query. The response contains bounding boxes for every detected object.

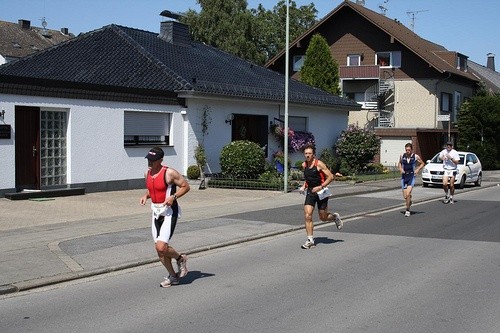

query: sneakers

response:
[333,213,343,230]
[177,255,189,277]
[301,239,316,249]
[160,274,180,288]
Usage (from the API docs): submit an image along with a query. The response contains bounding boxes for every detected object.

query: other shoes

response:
[450,198,454,204]
[404,211,410,217]
[408,195,412,206]
[444,196,450,204]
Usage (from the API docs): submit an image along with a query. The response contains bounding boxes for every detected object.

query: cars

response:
[421,149,484,189]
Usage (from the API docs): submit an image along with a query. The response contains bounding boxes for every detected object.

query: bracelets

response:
[321,185,323,189]
[451,158,453,160]
[173,195,177,199]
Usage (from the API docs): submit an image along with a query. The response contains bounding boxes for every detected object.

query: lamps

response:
[225,114,234,125]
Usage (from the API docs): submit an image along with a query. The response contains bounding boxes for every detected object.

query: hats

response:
[145,147,164,161]
[446,141,452,145]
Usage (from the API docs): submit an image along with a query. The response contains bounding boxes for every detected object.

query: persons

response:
[140,147,190,287]
[439,141,460,204]
[397,143,424,217]
[298,144,343,249]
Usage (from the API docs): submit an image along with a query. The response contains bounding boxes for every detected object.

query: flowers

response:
[270,122,316,153]
